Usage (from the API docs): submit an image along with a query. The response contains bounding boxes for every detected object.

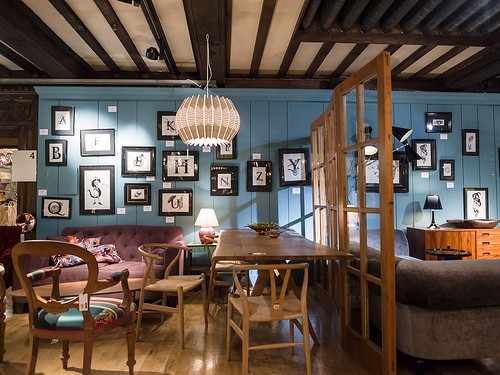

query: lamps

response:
[146,47,165,60]
[175,32,242,145]
[362,123,378,155]
[365,140,421,168]
[195,207,219,243]
[391,126,413,142]
[422,195,442,228]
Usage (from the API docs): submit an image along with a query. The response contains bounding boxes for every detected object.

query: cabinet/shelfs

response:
[407,226,500,261]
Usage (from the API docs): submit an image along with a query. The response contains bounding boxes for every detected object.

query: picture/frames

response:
[42,103,312,219]
[355,112,488,220]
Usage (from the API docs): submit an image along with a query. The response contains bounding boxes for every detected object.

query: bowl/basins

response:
[249,223,279,235]
[265,229,283,239]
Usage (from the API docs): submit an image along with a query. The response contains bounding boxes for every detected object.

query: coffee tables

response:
[12,278,157,300]
[426,249,472,261]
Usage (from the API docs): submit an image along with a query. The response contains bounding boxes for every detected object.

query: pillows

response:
[46,232,119,266]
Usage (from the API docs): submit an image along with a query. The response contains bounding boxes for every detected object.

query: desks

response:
[187,242,222,296]
[205,231,355,350]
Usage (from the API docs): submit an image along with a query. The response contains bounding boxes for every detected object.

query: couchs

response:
[0,225,22,287]
[11,225,183,314]
[329,229,500,375]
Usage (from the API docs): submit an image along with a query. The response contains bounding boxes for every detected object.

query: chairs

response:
[12,241,318,375]
